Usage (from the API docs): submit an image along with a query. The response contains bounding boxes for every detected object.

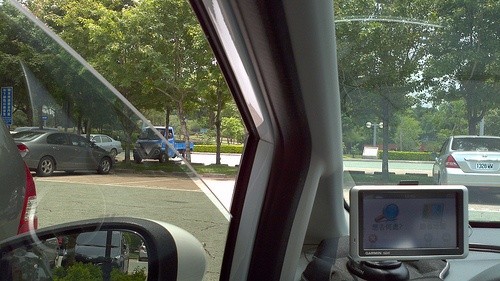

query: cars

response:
[0,112,39,244]
[26,237,61,269]
[72,132,123,158]
[9,126,62,135]
[60,231,130,274]
[10,129,116,176]
[135,241,148,262]
[430,135,500,195]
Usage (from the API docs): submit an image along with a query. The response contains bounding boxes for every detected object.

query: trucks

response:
[133,126,194,164]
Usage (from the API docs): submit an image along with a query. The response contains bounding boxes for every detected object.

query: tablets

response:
[349,185,469,260]
